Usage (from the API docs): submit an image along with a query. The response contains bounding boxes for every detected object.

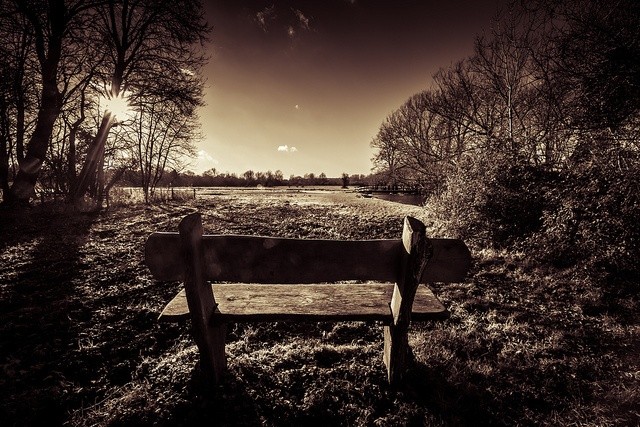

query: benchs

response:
[144,215,471,381]
